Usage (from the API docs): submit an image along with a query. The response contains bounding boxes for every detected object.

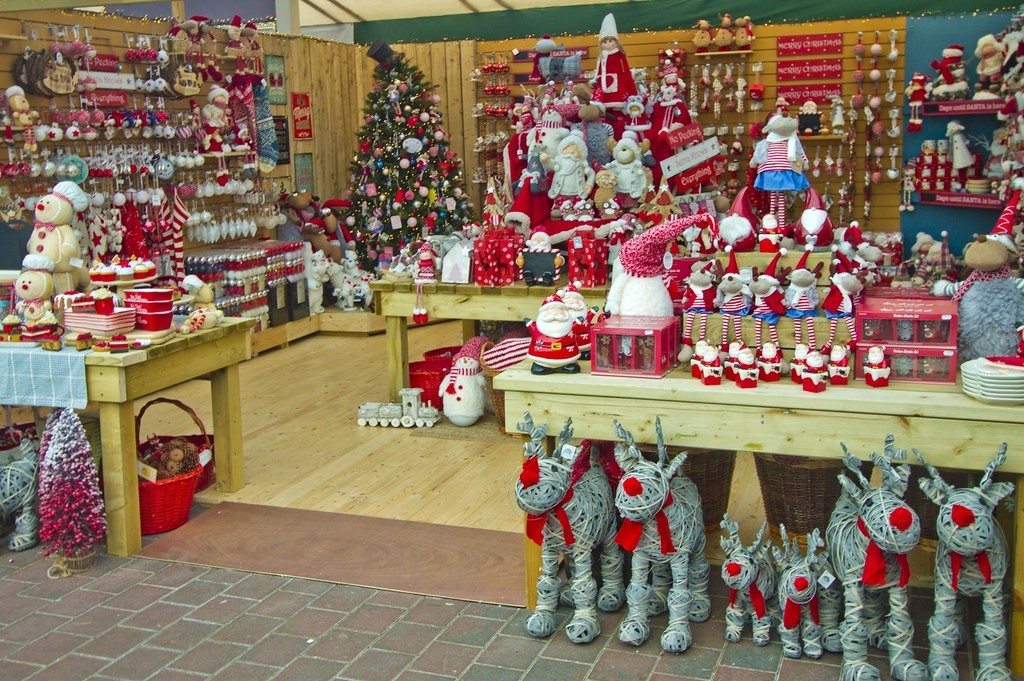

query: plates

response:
[961,358,1024,405]
[65,307,136,336]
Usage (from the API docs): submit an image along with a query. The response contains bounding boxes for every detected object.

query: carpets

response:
[410,393,583,446]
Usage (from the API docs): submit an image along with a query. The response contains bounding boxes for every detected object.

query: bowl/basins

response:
[967,177,990,193]
[123,288,173,330]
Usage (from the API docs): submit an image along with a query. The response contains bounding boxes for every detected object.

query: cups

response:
[921,139,936,153]
[937,140,949,154]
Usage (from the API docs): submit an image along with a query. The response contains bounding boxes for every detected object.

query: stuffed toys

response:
[172,15,264,186]
[277,189,378,315]
[904,72,930,131]
[606,213,708,368]
[438,335,493,426]
[15,254,58,329]
[26,181,88,294]
[931,176,1024,359]
[183,275,224,325]
[501,12,754,227]
[983,127,1012,180]
[975,33,1005,81]
[175,309,225,335]
[410,241,442,324]
[677,208,956,374]
[6,85,39,151]
[898,157,917,211]
[749,97,809,236]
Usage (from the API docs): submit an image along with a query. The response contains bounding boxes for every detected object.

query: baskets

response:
[98,397,216,535]
[423,346,463,361]
[753,452,865,552]
[409,361,450,410]
[480,342,523,438]
[634,442,737,533]
[891,463,984,540]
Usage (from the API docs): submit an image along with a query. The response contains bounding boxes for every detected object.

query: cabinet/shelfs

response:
[906,95,1024,211]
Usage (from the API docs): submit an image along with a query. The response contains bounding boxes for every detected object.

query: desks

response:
[0,315,262,556]
[492,347,1024,673]
[373,269,614,405]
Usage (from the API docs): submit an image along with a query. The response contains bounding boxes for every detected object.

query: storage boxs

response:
[664,256,708,303]
[591,315,684,376]
[856,287,959,382]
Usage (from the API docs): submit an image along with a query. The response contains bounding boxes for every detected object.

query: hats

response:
[367,39,405,72]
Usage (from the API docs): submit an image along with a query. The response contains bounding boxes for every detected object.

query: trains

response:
[357,387,442,427]
